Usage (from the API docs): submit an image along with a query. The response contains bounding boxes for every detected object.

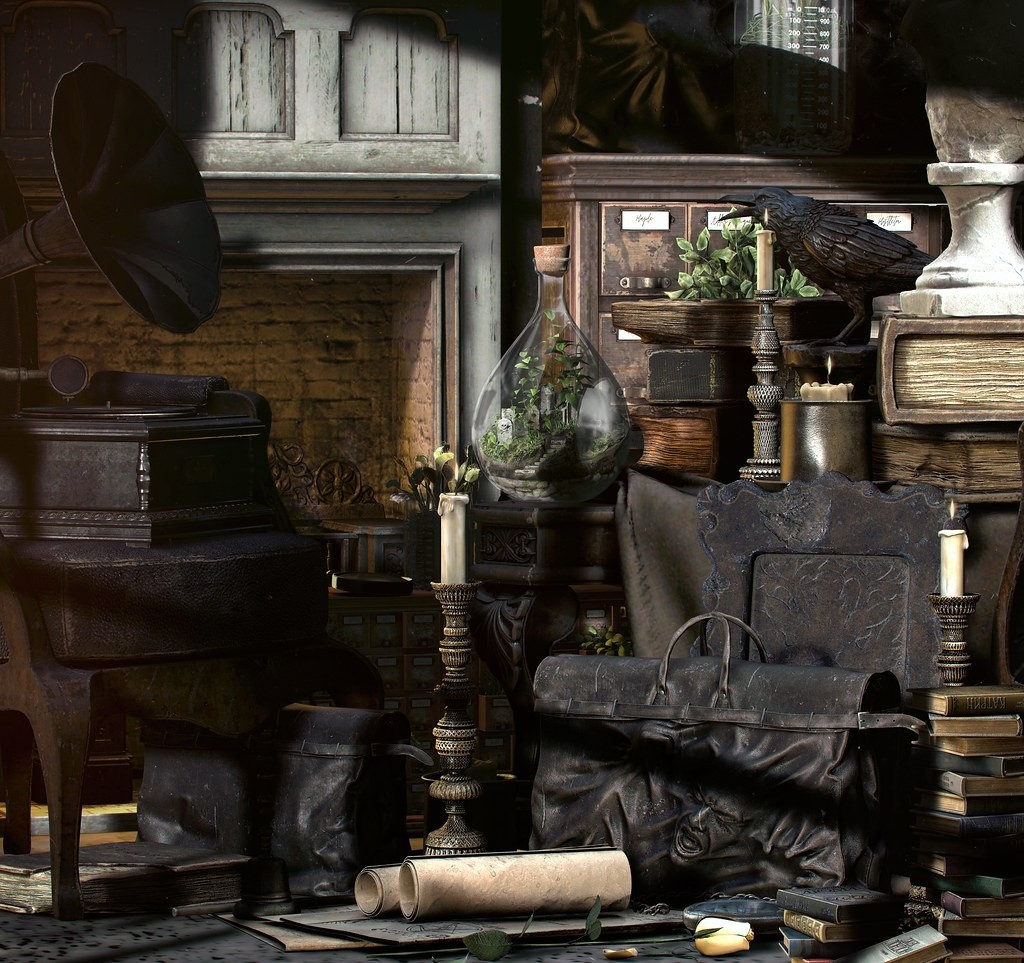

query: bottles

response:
[471,244,632,502]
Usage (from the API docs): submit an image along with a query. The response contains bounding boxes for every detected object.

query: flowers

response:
[386,443,481,512]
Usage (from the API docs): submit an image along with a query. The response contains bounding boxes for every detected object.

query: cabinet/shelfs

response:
[328,584,630,838]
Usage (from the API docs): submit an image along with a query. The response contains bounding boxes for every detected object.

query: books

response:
[871,421,1024,503]
[0,841,252,915]
[627,404,748,482]
[645,344,740,404]
[778,685,1024,963]
[612,296,856,348]
[875,314,1024,428]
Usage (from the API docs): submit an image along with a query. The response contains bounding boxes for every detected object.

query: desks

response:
[0,531,386,922]
[538,152,943,414]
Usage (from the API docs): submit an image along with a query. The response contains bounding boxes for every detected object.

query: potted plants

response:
[470,243,632,504]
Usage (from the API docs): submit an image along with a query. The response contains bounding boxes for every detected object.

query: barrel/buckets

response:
[778,397,874,483]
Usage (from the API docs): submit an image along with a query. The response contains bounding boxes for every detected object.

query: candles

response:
[437,462,470,583]
[937,499,969,597]
[800,353,855,401]
[755,207,777,290]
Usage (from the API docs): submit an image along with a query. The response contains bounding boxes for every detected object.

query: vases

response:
[409,509,474,591]
